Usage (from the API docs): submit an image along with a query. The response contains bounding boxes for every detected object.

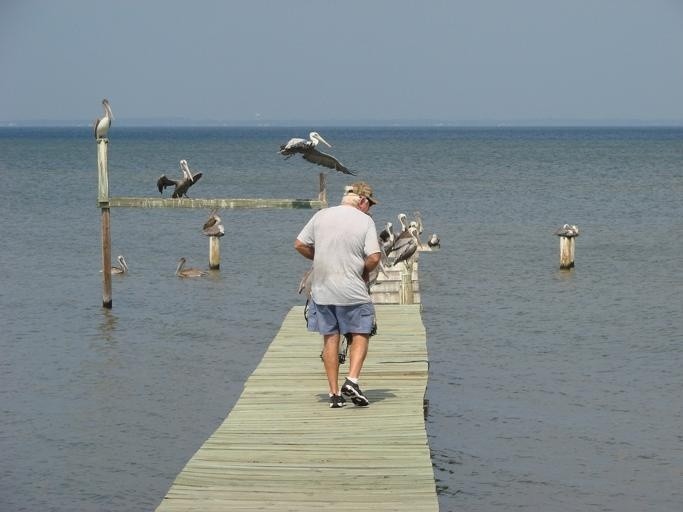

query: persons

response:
[294,181,381,408]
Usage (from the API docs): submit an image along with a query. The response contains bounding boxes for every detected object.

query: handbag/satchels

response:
[295,270,313,302]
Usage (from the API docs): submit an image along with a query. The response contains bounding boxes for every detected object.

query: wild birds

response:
[98,255,129,275]
[202,204,226,237]
[425,231,440,250]
[157,158,202,199]
[376,209,424,269]
[553,223,579,238]
[276,131,358,177]
[93,97,114,145]
[174,257,209,278]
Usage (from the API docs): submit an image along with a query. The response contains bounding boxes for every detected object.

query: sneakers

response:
[328,379,370,408]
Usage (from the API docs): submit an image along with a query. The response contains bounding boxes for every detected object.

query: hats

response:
[346,180,381,206]
